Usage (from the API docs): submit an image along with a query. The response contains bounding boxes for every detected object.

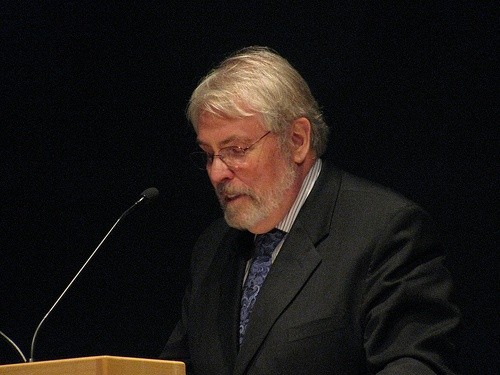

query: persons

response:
[160,46,488,375]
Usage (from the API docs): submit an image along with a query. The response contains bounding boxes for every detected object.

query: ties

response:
[238,227,288,354]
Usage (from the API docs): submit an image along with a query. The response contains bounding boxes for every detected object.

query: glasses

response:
[190,124,288,171]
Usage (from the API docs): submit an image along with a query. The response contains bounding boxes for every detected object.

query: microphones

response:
[29,188,160,363]
